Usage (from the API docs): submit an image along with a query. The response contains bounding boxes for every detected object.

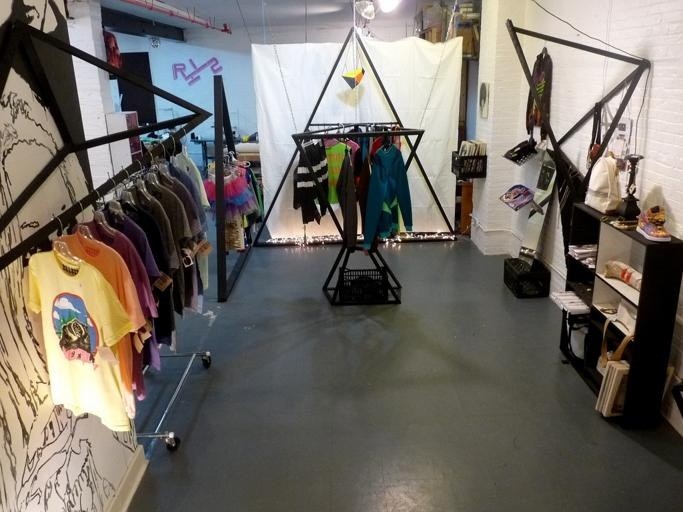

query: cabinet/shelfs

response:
[456,175,474,238]
[557,199,682,432]
[105,110,144,198]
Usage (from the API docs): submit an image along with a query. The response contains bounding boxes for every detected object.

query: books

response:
[454,138,487,173]
[408,0,480,58]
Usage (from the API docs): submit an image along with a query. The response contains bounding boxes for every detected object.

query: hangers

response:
[295,121,403,160]
[535,37,551,61]
[205,151,252,178]
[55,121,190,273]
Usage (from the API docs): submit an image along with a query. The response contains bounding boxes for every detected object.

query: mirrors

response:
[517,148,558,270]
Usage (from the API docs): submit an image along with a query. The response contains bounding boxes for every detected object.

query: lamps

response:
[355,0,404,19]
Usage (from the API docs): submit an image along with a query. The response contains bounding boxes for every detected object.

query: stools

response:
[236,152,263,197]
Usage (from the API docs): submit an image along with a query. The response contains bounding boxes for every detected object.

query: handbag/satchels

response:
[587,144,600,170]
[601,298,638,368]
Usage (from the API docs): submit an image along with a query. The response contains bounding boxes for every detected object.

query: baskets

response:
[452,151,487,179]
[339,269,388,304]
[504,257,551,299]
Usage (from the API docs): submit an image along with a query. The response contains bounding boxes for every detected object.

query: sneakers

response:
[636,206,671,242]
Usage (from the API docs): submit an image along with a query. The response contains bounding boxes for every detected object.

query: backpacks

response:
[585,157,620,214]
[568,314,603,368]
